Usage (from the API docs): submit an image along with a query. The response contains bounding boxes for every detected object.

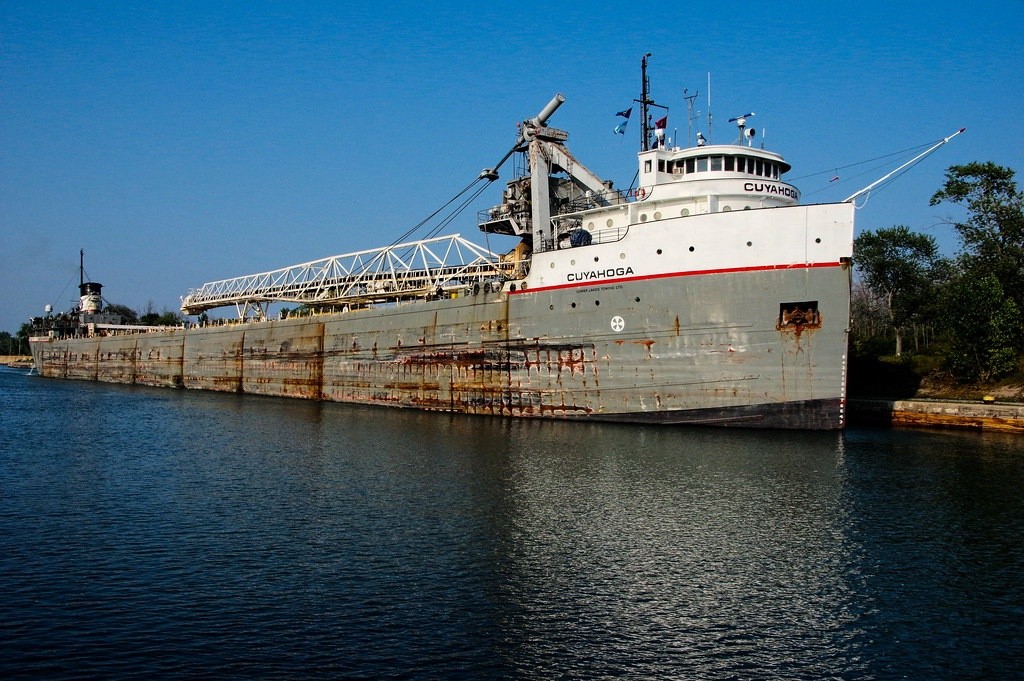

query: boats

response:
[8,356,37,369]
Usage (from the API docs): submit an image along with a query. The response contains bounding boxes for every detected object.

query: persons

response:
[343,304,349,313]
[436,284,445,301]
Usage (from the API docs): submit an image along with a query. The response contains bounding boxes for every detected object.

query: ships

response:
[27,47,966,439]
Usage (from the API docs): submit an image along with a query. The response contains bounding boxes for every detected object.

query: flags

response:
[613,120,629,135]
[652,133,665,149]
[655,116,666,128]
[615,107,632,119]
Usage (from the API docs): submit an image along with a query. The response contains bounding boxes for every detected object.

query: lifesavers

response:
[635,186,646,201]
